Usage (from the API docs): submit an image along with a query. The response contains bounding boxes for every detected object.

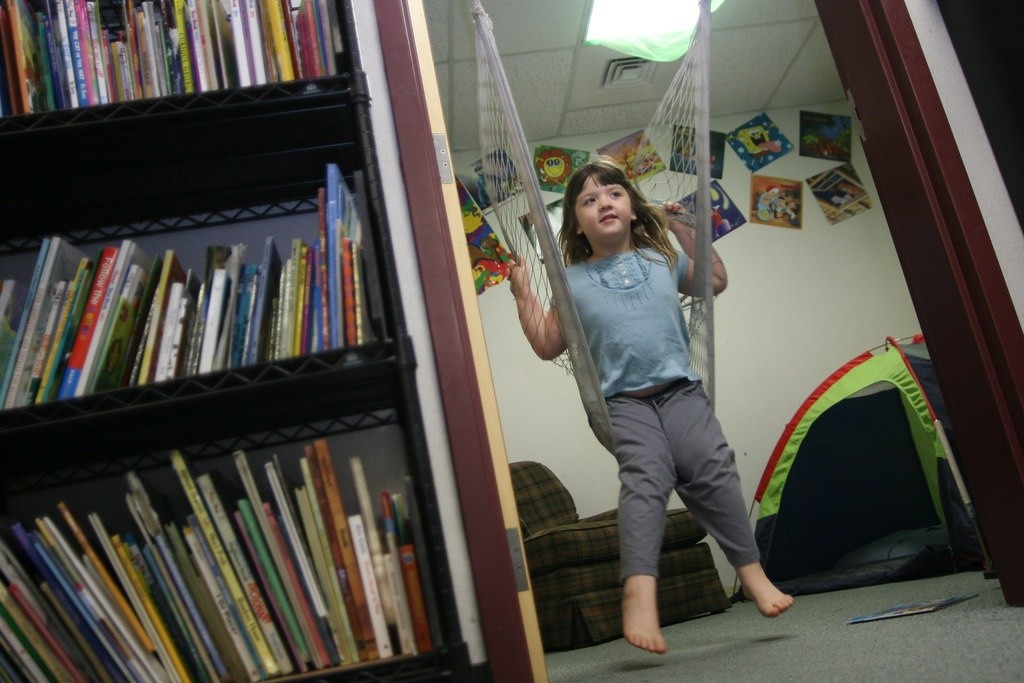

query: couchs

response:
[509,460,733,653]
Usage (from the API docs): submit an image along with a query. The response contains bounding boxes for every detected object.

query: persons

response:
[505,155,792,655]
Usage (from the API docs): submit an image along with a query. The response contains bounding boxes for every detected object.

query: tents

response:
[730,333,992,601]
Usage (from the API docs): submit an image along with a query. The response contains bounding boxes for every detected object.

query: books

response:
[0,0,446,683]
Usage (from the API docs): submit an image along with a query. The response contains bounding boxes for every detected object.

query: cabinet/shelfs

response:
[0,0,475,683]
